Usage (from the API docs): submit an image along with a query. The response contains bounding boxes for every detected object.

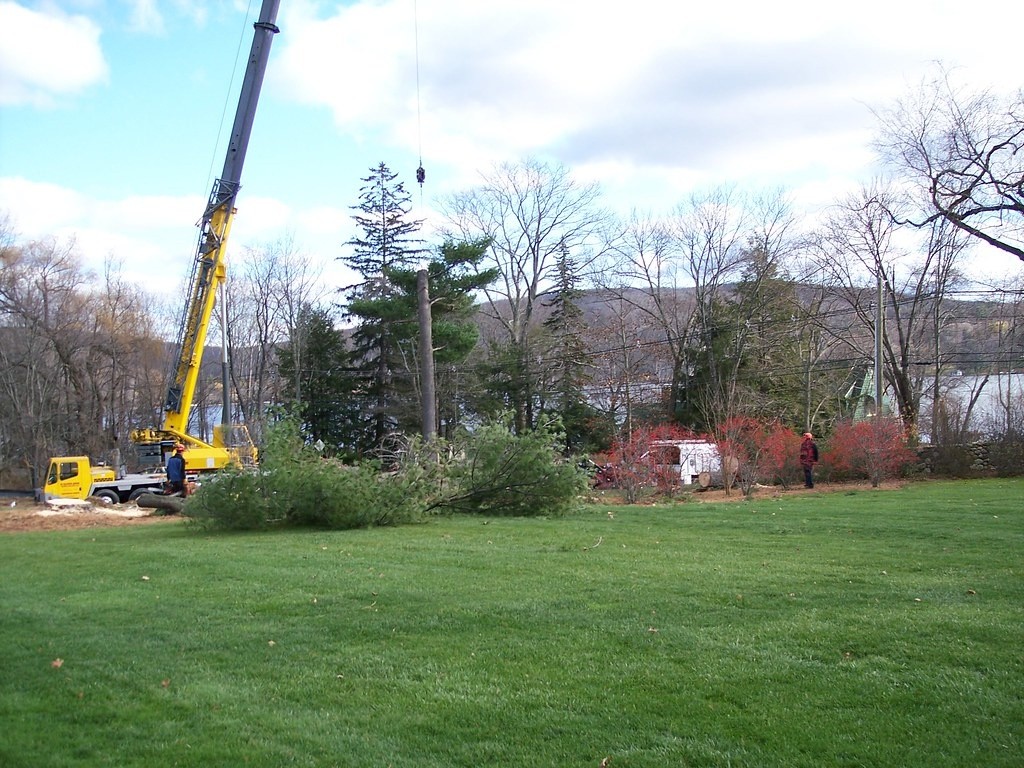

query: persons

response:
[799,432,819,489]
[166,444,186,498]
[591,462,616,490]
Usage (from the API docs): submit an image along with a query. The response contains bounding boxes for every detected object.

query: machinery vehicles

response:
[45,0,426,504]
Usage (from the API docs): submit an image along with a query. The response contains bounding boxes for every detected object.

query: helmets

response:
[805,433,813,438]
[176,444,186,451]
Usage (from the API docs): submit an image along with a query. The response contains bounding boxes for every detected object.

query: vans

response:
[646,437,722,487]
